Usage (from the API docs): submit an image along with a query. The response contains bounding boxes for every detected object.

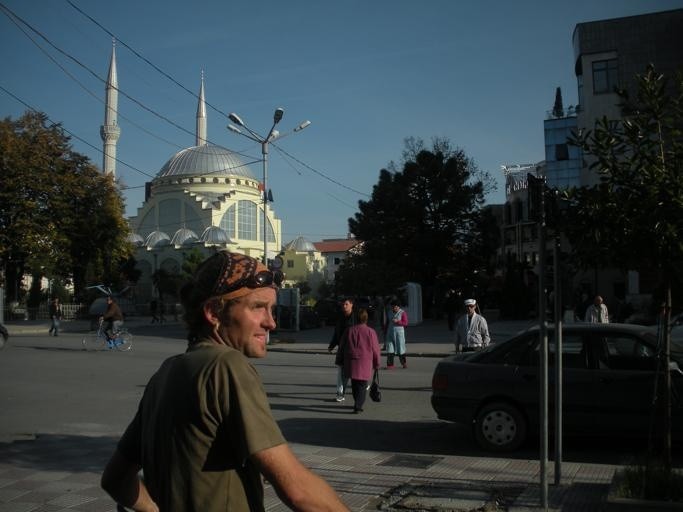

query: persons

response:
[148,296,159,324]
[157,298,166,324]
[584,295,610,323]
[338,306,383,414]
[47,297,61,337]
[326,296,357,402]
[384,298,409,370]
[100,250,352,511]
[98,294,124,341]
[452,298,491,353]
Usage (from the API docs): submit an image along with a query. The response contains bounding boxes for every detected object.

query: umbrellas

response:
[552,84,567,120]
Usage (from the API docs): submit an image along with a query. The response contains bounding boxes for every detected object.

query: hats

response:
[464,299,477,305]
[186,250,279,303]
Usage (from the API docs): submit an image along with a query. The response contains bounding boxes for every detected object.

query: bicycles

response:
[81,317,132,352]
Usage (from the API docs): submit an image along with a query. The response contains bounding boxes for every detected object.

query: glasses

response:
[221,270,284,294]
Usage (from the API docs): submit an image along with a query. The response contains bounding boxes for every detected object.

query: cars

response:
[0,324,8,351]
[430,322,683,453]
[272,297,377,328]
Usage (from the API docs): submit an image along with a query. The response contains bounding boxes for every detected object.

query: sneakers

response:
[336,364,409,414]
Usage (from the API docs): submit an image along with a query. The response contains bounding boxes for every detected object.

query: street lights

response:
[225,108,311,345]
